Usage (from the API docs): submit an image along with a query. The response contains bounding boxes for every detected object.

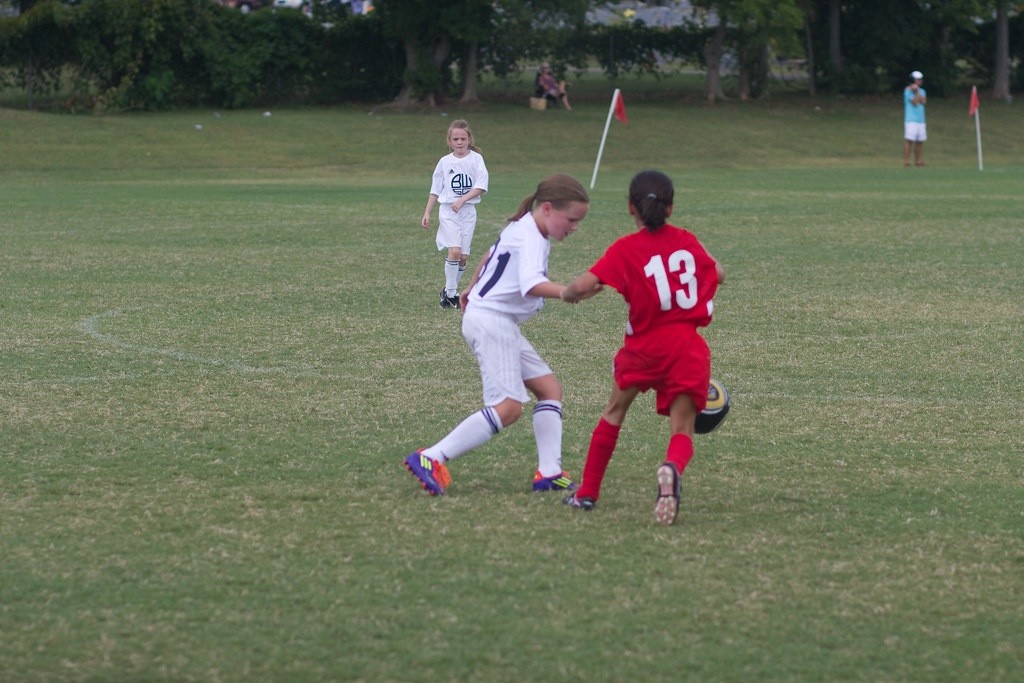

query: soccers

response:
[693,378,732,434]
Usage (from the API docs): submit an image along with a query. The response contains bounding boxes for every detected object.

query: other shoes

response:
[905,161,927,166]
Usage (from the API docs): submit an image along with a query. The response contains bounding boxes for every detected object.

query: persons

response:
[399,174,606,494]
[536,61,573,111]
[556,167,724,527]
[416,117,489,307]
[900,70,929,166]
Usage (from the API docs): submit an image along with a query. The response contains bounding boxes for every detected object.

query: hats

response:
[912,71,924,80]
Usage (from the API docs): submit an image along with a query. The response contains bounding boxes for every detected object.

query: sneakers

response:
[653,462,682,526]
[562,491,596,510]
[447,296,461,309]
[438,287,447,309]
[533,471,579,492]
[403,448,446,495]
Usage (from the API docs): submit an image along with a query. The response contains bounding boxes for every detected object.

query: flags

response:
[614,93,629,124]
[968,87,980,116]
